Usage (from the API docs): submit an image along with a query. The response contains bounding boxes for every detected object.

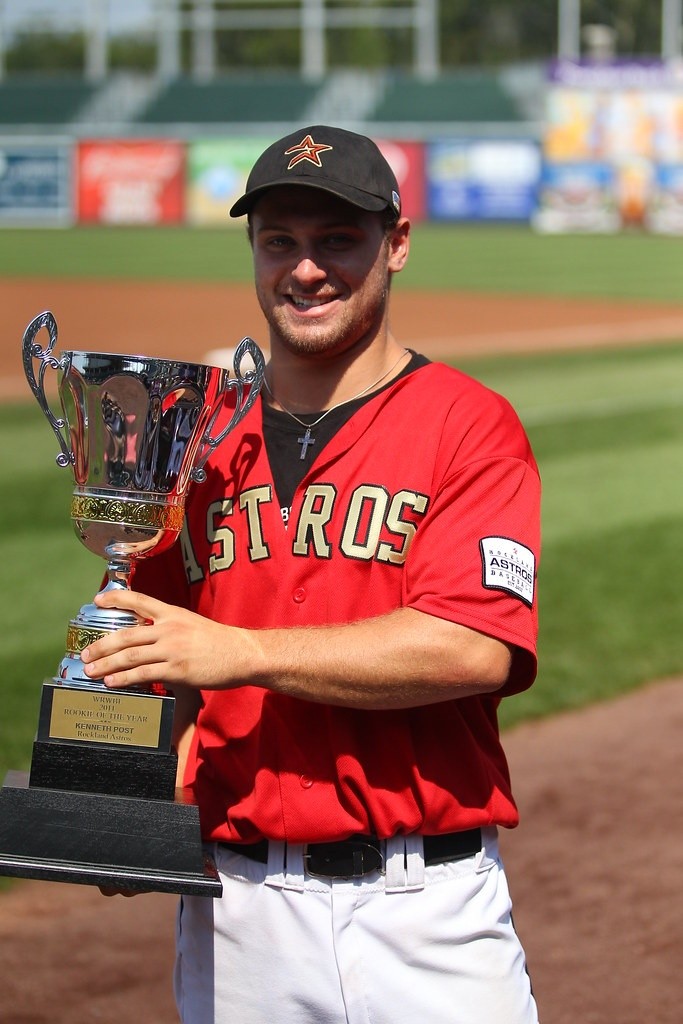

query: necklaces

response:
[261,349,411,461]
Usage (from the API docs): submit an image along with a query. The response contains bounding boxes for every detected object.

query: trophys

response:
[2,312,272,895]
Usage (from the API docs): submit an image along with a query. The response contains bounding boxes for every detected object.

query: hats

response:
[228,125,401,221]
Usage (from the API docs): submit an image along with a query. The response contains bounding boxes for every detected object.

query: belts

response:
[214,828,482,879]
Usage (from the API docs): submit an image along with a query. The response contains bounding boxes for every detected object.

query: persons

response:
[81,124,546,1024]
